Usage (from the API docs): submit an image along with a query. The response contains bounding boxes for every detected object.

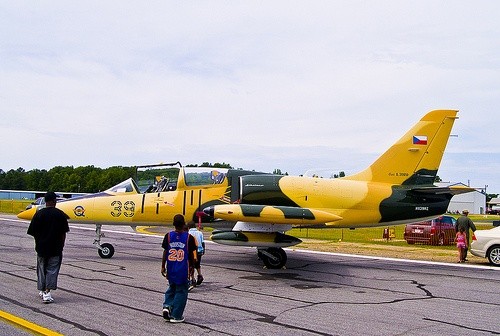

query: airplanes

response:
[16,107,486,269]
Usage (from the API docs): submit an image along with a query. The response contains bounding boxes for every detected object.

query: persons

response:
[455,210,476,261]
[160,215,198,322]
[27,192,71,303]
[188,221,206,289]
[453,226,468,262]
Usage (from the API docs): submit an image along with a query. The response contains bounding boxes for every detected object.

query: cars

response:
[25,197,66,210]
[470,224,500,266]
[403,215,457,247]
[488,210,500,215]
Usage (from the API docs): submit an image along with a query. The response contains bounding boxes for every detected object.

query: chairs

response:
[144,177,170,193]
[213,172,225,183]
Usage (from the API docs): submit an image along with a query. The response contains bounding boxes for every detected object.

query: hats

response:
[45,191,60,202]
[463,210,469,214]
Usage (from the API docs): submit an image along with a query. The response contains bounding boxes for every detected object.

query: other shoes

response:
[191,279,197,286]
[187,282,193,291]
[197,275,203,285]
[457,258,468,263]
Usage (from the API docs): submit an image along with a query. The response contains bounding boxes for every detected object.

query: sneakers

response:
[162,306,170,321]
[170,316,185,322]
[39,290,44,298]
[42,291,53,303]
